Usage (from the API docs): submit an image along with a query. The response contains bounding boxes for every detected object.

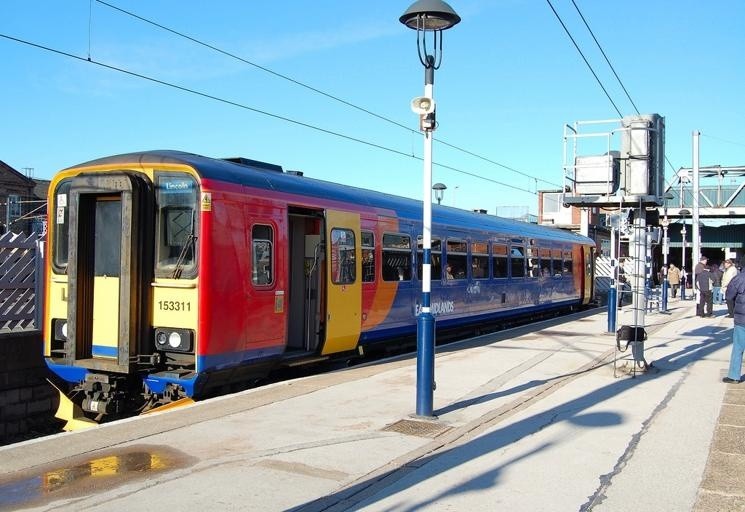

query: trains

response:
[43,149,597,433]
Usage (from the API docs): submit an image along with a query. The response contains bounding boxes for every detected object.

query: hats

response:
[700,256,732,270]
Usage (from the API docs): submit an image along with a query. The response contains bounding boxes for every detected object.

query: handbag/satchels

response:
[616,325,648,353]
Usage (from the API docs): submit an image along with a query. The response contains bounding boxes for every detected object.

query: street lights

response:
[699,221,704,258]
[662,193,674,310]
[399,1,460,416]
[679,210,690,300]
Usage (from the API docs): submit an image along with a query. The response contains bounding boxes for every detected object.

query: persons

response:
[613,257,627,311]
[659,255,745,318]
[446,263,455,280]
[722,255,745,384]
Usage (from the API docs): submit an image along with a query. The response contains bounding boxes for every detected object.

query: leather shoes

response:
[723,377,740,384]
